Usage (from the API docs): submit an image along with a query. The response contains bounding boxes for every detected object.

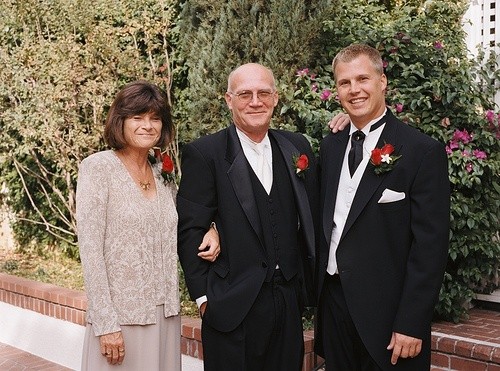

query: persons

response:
[176,63,352,371]
[314,44,451,371]
[76,83,221,371]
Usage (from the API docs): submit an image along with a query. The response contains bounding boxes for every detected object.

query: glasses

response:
[228,89,275,102]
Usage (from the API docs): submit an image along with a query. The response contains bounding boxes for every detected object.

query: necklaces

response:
[119,154,151,190]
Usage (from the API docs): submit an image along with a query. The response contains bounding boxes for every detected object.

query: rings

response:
[119,349,124,352]
[105,352,112,355]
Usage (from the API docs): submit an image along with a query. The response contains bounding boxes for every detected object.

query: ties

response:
[348,111,388,179]
[250,141,271,194]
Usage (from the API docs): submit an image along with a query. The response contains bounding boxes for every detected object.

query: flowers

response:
[292,153,310,179]
[371,144,403,174]
[154,149,174,186]
